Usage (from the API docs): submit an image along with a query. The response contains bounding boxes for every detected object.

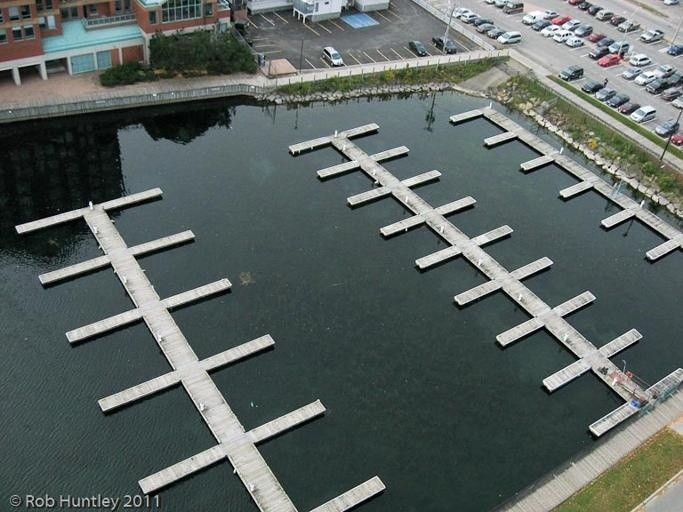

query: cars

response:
[520,0,682,148]
[451,0,524,44]
[408,40,426,57]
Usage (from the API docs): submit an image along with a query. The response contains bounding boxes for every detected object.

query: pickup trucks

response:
[431,35,457,53]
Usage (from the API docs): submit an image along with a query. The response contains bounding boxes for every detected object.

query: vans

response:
[321,46,343,66]
